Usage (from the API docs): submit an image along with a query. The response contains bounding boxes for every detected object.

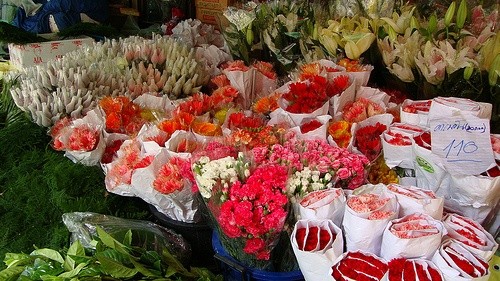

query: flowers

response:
[0,0,500,281]
